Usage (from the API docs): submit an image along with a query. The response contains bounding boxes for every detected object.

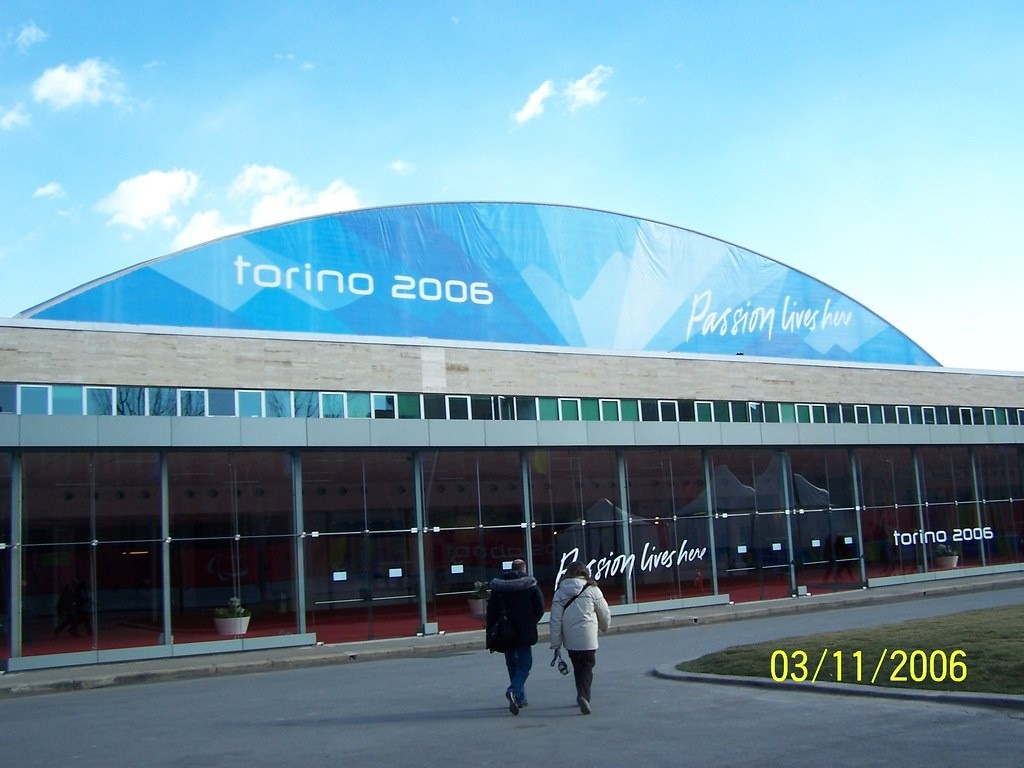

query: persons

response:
[486,559,544,716]
[549,561,611,714]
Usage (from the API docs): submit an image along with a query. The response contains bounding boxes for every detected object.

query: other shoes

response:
[579,696,591,714]
[506,691,520,715]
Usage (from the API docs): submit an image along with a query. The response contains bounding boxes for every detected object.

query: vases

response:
[935,556,959,568]
[216,617,250,635]
[468,598,488,615]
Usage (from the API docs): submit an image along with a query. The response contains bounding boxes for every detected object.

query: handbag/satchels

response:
[550,649,571,676]
[485,617,515,653]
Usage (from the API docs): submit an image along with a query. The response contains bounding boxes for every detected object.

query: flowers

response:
[214,595,253,618]
[468,578,492,600]
[935,543,959,556]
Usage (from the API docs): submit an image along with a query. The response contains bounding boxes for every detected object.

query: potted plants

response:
[797,583,808,595]
